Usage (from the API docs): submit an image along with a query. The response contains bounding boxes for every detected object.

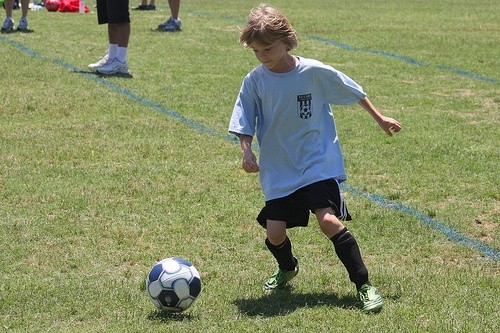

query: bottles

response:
[79,0,85,14]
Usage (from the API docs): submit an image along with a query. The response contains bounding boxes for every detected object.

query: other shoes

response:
[133,4,155,10]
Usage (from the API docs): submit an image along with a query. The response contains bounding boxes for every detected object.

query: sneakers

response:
[357,284,383,312]
[88,53,111,72]
[263,256,300,291]
[97,58,128,75]
[1,20,13,30]
[158,16,181,32]
[17,19,28,30]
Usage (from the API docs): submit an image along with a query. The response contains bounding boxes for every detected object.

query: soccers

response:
[145,257,202,312]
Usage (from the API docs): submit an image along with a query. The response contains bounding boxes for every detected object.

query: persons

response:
[158,0,181,31]
[135,0,156,10]
[228,7,402,312]
[1,0,29,33]
[88,0,130,74]
